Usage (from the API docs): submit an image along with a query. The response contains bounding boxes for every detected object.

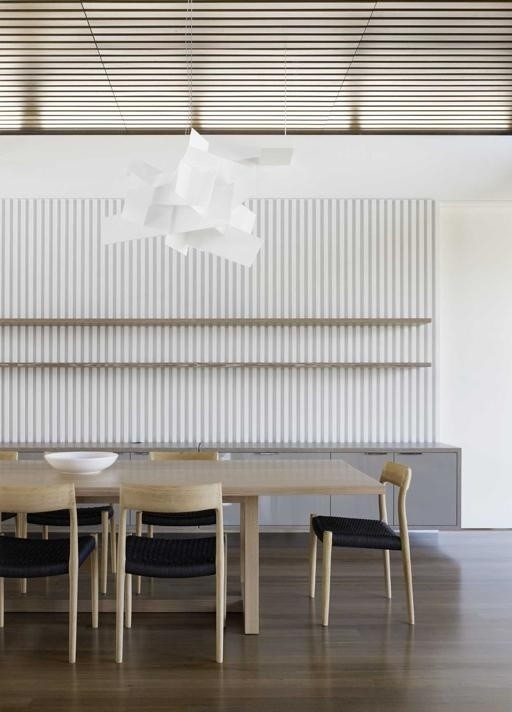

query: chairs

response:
[0,451,21,537]
[116,481,228,664]
[0,482,99,664]
[309,460,415,627]
[26,503,117,594]
[134,452,223,595]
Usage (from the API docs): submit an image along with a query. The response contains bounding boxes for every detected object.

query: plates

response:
[43,451,119,475]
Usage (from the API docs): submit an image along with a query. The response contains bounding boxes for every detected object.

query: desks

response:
[1,459,385,635]
